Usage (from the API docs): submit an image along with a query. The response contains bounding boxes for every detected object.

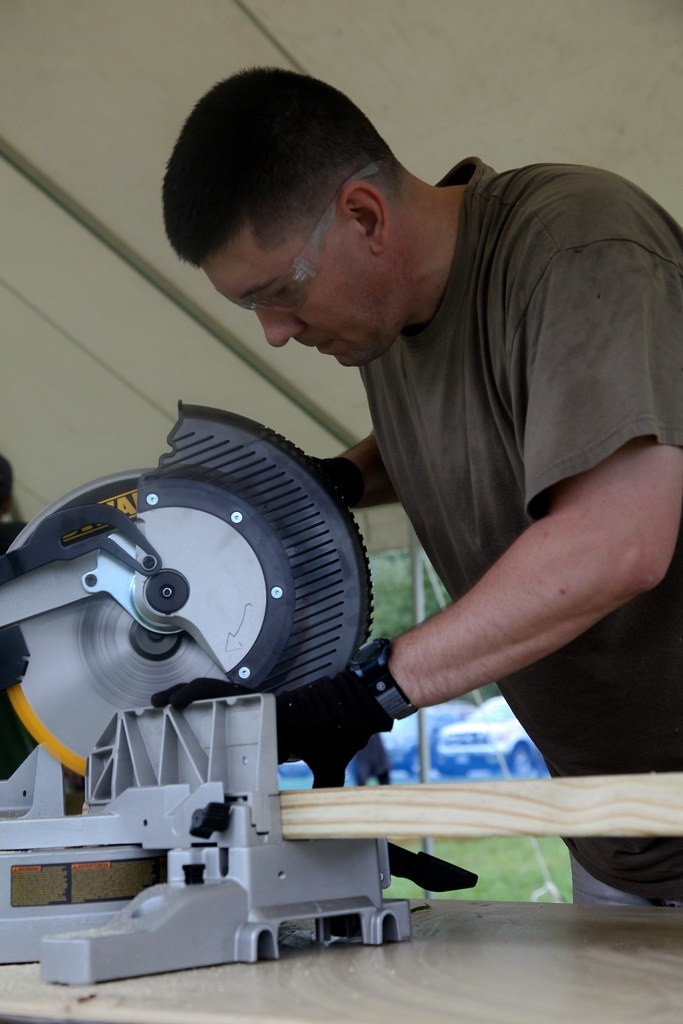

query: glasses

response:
[225,163,378,313]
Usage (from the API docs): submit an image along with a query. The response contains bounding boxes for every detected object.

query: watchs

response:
[350,638,419,720]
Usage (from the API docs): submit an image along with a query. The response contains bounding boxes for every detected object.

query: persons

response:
[162,64,683,911]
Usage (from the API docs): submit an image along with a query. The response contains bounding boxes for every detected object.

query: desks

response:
[0,897,683,1024]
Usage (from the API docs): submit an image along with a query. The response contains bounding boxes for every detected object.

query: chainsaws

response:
[0,398,479,987]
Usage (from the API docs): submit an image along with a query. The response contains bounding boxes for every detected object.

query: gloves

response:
[151,669,393,788]
